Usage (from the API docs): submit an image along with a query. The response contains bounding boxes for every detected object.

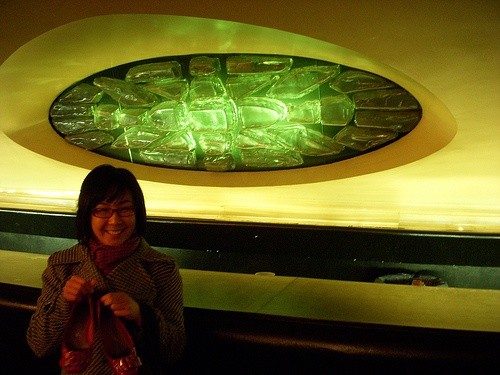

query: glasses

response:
[91,205,136,219]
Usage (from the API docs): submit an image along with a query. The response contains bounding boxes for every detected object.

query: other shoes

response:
[96,300,142,375]
[59,292,95,375]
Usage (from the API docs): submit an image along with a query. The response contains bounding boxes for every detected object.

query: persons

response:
[25,164,187,375]
